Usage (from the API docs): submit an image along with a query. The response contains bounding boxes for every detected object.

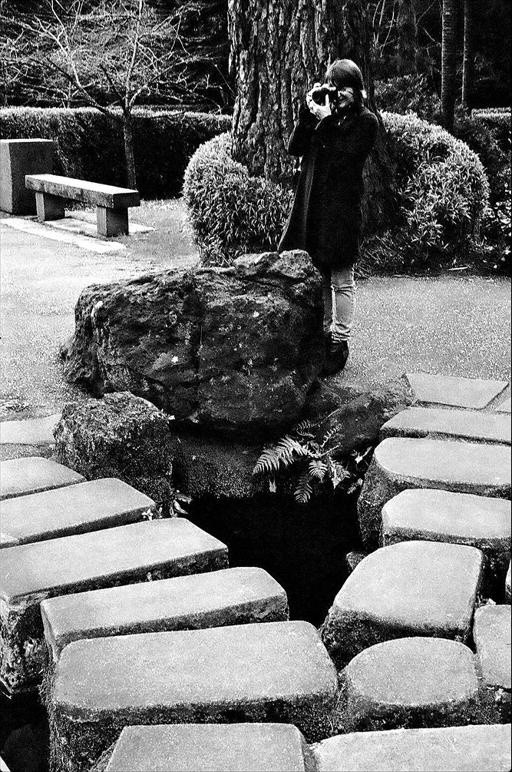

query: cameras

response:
[311,87,339,105]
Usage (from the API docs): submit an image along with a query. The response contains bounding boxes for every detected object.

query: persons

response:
[278,59,381,376]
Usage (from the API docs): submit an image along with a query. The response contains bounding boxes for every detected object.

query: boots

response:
[317,331,348,378]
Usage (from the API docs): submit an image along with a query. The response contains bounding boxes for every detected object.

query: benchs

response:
[24,172,141,236]
